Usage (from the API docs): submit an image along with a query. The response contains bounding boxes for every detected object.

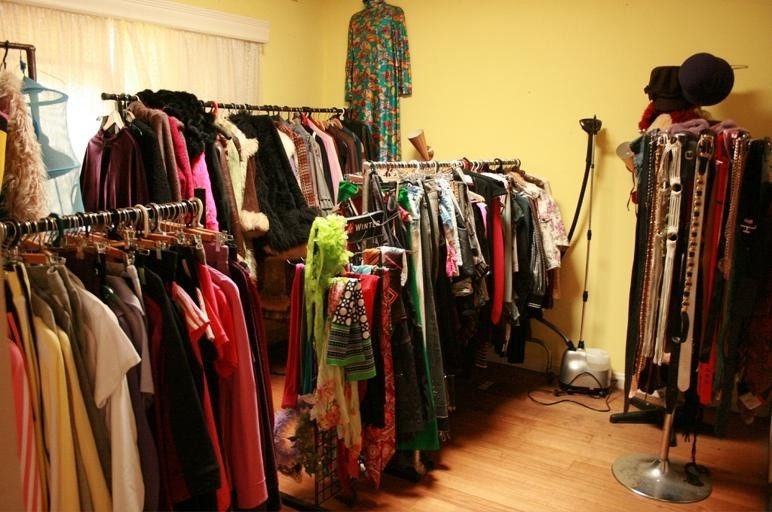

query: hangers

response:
[0,40,10,71]
[0,198,234,271]
[285,157,520,281]
[96,92,352,140]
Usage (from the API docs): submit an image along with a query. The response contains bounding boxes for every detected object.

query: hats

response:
[678,53,733,106]
[644,66,694,112]
